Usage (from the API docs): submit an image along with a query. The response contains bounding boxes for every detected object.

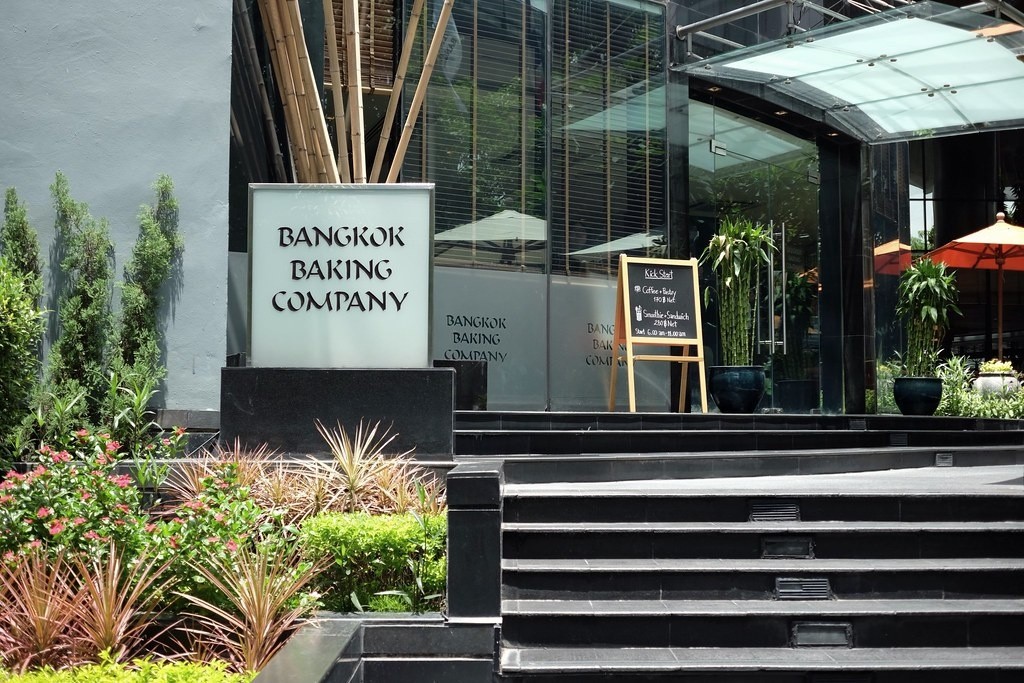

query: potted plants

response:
[696,216,778,414]
[890,259,962,416]
[974,359,1021,397]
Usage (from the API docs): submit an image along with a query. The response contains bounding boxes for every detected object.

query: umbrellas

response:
[873,239,912,275]
[911,213,1024,363]
[800,267,818,283]
[563,232,665,264]
[433,209,547,265]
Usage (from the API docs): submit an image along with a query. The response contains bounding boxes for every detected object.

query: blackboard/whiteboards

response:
[618,256,705,343]
